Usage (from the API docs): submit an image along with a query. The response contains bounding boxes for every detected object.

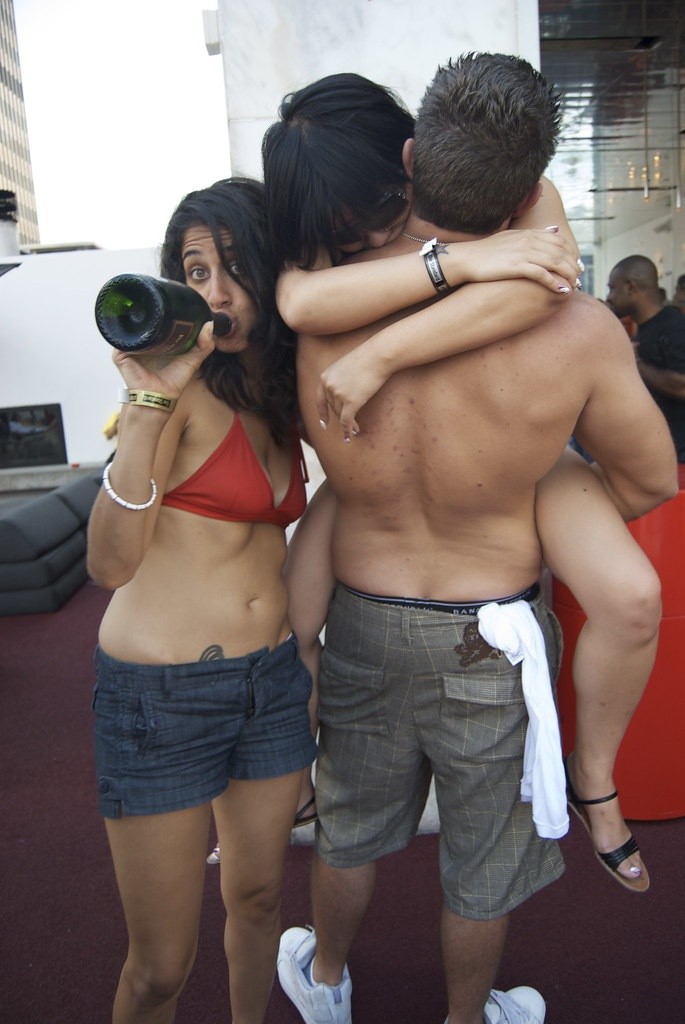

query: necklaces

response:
[401,232,446,246]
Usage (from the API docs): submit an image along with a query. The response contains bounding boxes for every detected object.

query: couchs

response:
[0,476,99,617]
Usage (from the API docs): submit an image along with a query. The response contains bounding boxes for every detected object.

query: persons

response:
[206,53,685,1024]
[86,177,313,1024]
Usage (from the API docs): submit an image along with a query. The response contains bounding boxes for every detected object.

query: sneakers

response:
[277,923,352,1024]
[444,986,546,1024]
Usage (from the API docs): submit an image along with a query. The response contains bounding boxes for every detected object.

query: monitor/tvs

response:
[0,403,68,470]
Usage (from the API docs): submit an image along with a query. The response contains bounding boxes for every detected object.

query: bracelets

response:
[102,461,156,510]
[419,237,452,295]
[115,389,178,413]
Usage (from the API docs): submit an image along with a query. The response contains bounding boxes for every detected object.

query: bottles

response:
[94,273,231,353]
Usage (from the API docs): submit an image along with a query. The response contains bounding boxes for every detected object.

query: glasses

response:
[324,183,410,247]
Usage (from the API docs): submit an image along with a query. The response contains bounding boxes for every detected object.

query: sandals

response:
[564,756,650,892]
[206,794,317,864]
